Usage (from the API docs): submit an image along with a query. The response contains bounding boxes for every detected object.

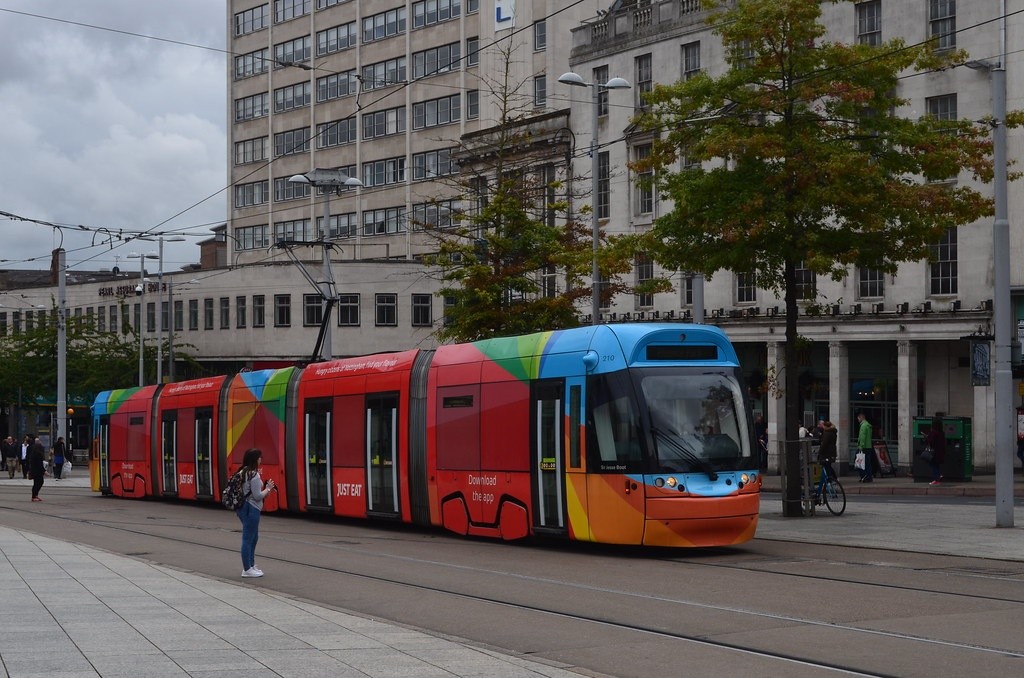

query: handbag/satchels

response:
[854,450,865,470]
[923,441,934,461]
[62,460,72,474]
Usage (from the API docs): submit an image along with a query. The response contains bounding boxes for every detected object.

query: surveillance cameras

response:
[135,286,143,296]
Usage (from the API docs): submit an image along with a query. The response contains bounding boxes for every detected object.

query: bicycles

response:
[800,455,847,516]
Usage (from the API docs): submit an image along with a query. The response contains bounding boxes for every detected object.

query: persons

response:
[925,419,946,484]
[856,414,873,483]
[817,422,838,481]
[798,420,825,444]
[53,437,66,480]
[680,423,713,435]
[236,449,275,576]
[1,433,34,479]
[654,401,680,436]
[25,436,46,502]
[755,416,768,472]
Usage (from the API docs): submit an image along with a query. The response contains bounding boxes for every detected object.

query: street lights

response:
[138,236,187,384]
[127,251,159,387]
[289,168,363,361]
[557,72,632,327]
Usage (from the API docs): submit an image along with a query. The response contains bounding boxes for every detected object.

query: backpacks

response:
[221,466,252,511]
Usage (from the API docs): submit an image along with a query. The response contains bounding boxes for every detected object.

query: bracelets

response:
[267,488,270,492]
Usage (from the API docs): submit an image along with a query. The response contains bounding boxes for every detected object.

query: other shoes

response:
[31,497,41,501]
[929,480,940,485]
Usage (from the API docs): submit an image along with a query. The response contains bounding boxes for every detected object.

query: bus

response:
[88,239,763,550]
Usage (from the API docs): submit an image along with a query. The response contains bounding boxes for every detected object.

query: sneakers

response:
[241,566,263,578]
